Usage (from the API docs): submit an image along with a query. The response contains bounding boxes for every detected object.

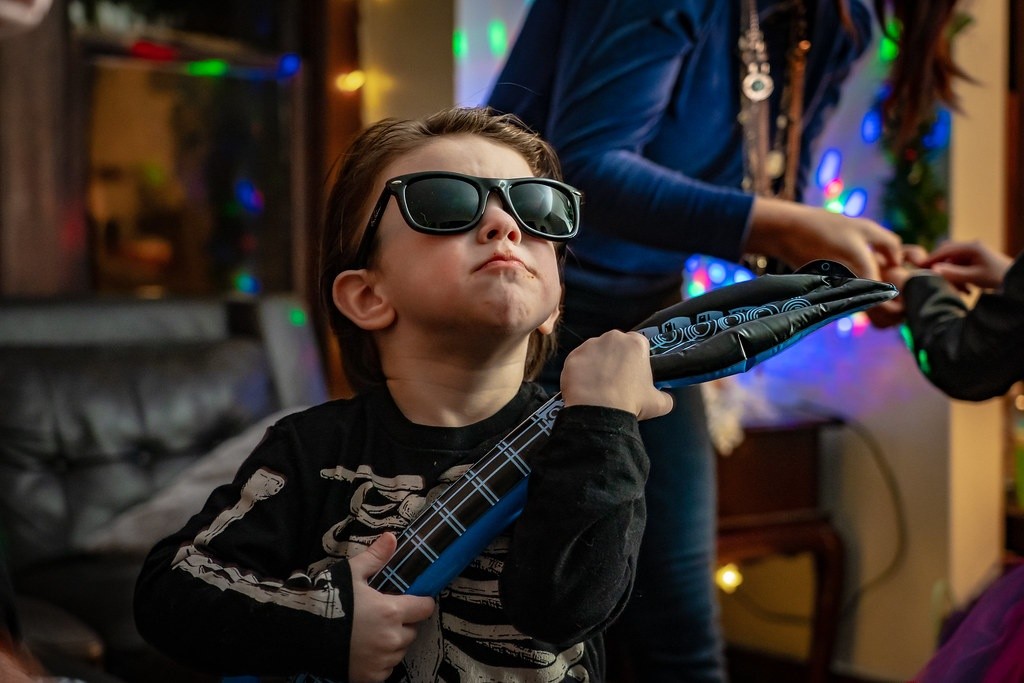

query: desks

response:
[708,403,859,678]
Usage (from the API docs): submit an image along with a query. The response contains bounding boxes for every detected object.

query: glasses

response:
[352,169,583,270]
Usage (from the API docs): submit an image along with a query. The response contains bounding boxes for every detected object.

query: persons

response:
[132,107,673,682]
[868,238,1023,403]
[482,0,980,681]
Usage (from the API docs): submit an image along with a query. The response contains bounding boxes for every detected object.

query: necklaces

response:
[739,0,810,276]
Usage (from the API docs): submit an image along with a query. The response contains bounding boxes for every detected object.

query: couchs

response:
[3,340,301,682]
